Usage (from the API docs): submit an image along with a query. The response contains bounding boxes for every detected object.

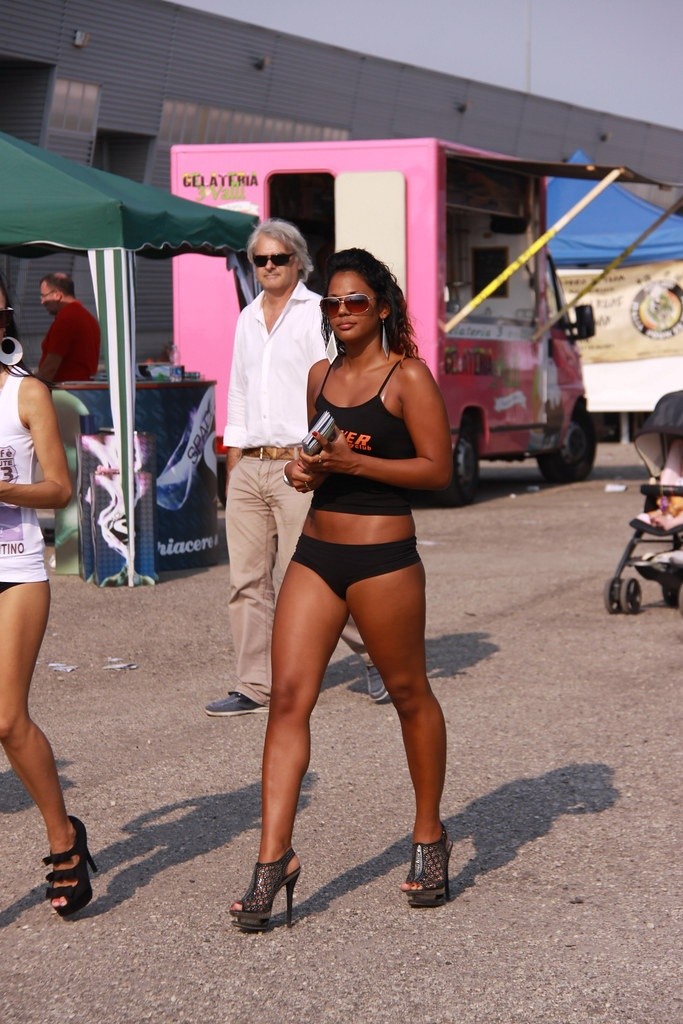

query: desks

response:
[47,376,220,585]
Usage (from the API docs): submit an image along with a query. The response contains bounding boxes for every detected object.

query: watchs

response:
[282,461,292,487]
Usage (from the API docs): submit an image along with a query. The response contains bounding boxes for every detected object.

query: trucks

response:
[169,141,683,507]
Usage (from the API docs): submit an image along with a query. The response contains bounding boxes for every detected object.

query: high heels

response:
[43,815,98,916]
[404,823,453,907]
[230,847,300,931]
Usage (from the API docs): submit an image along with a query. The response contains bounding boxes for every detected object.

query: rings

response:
[318,454,322,463]
[305,481,312,491]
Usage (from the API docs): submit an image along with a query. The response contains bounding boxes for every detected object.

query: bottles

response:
[169,344,181,382]
[444,344,494,378]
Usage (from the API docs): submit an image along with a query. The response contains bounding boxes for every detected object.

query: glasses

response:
[252,252,296,268]
[0,307,15,328]
[40,289,56,300]
[319,294,378,317]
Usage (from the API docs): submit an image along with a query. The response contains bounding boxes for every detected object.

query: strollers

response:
[603,392,683,616]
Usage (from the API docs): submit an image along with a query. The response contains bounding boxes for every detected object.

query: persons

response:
[230,256,455,931]
[204,219,389,718]
[0,278,99,917]
[33,272,100,384]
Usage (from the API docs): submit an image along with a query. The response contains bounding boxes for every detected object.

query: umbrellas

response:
[546,150,683,265]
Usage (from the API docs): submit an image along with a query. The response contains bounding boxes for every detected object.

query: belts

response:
[241,445,302,461]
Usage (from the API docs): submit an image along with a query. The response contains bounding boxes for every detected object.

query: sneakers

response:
[366,664,388,701]
[203,692,270,716]
